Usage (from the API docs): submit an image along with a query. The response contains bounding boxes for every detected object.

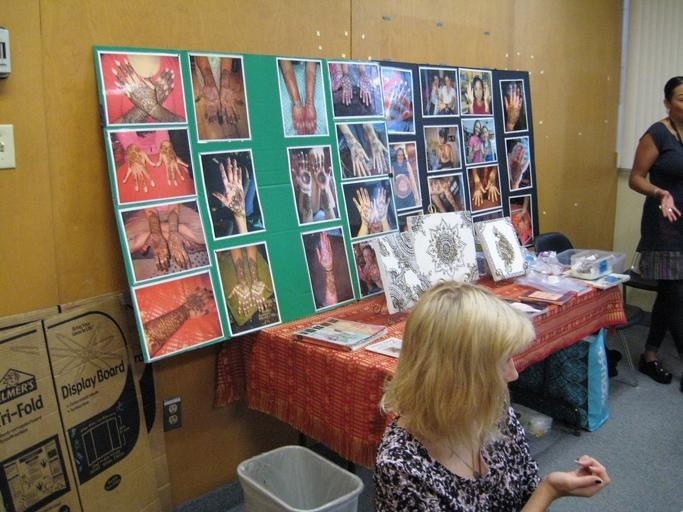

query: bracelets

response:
[654,188,659,199]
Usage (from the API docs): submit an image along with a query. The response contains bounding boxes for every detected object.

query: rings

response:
[667,208,672,213]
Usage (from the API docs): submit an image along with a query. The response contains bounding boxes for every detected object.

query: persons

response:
[278,58,338,311]
[109,56,187,125]
[375,280,609,510]
[193,56,274,317]
[423,70,535,245]
[138,205,195,271]
[328,63,420,295]
[142,287,214,359]
[122,139,190,193]
[628,76,683,391]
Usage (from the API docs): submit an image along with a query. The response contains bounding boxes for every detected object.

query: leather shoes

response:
[638,354,672,383]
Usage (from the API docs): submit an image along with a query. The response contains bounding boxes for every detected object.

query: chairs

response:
[623,235,658,314]
[534,233,645,388]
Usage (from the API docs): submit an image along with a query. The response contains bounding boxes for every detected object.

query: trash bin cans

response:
[235,444,364,512]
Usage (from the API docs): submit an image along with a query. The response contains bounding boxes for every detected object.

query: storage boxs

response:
[556,249,582,273]
[569,249,616,281]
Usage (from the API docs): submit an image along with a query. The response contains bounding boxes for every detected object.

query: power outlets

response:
[163,397,180,431]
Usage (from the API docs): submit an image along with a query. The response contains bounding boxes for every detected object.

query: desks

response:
[213,270,630,471]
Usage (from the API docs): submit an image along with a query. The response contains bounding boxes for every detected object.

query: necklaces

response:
[668,119,682,148]
[445,444,478,480]
